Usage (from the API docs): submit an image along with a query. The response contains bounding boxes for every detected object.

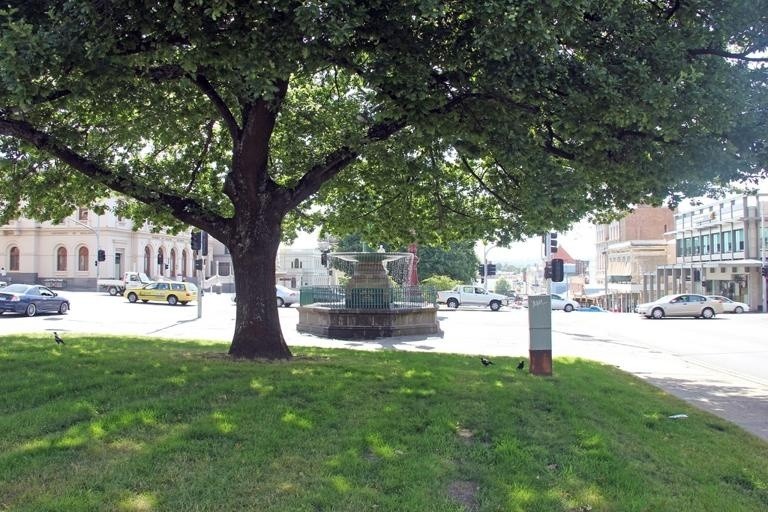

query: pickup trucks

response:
[97,271,149,296]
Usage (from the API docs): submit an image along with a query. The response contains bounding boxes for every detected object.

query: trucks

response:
[435,285,505,312]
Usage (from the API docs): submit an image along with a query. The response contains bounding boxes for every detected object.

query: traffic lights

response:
[551,232,558,252]
[552,258,563,282]
[478,262,496,275]
[190,228,201,250]
[98,249,105,261]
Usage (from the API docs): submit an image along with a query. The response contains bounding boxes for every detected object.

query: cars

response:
[634,293,726,319]
[520,293,580,312]
[123,280,200,306]
[1,282,72,318]
[232,283,301,308]
[705,294,752,314]
[310,284,345,304]
